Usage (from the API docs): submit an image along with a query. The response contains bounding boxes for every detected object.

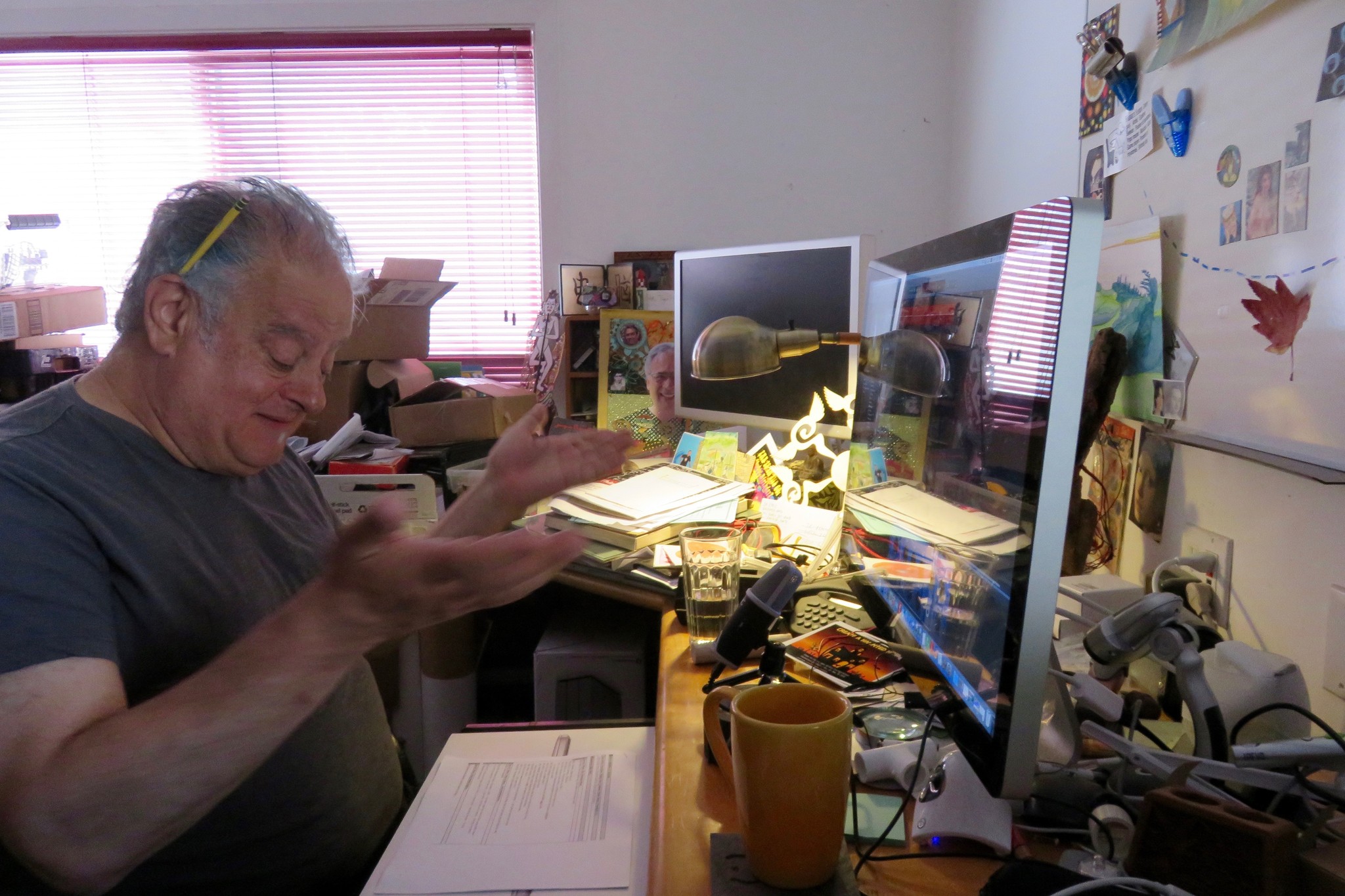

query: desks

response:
[556,571,1031,896]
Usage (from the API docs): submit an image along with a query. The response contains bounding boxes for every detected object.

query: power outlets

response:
[1181,522,1233,630]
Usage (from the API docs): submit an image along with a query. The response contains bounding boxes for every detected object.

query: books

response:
[543,431,757,570]
[298,412,366,467]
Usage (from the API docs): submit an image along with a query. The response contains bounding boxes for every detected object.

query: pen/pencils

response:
[176,194,250,279]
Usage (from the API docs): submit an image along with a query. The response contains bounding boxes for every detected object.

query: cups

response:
[703,682,852,884]
[923,542,1000,660]
[678,525,742,647]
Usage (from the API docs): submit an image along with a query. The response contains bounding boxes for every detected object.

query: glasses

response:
[647,372,674,383]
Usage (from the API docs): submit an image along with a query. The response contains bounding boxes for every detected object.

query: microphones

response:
[712,560,802,670]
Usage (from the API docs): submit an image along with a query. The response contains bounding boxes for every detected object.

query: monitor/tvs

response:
[833,192,1105,786]
[673,236,861,439]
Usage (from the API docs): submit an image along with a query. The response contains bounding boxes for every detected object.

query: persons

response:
[679,450,691,466]
[0,176,635,896]
[623,325,641,346]
[1153,383,1164,416]
[611,370,627,393]
[610,343,700,455]
[1135,436,1171,525]
[1165,384,1183,420]
[1222,154,1237,182]
[1248,165,1277,238]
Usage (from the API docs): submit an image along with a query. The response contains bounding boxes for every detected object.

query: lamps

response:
[859,329,945,398]
[689,316,862,380]
[1083,591,1229,806]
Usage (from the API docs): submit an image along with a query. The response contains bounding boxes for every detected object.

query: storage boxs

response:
[0,256,539,525]
[533,615,648,719]
